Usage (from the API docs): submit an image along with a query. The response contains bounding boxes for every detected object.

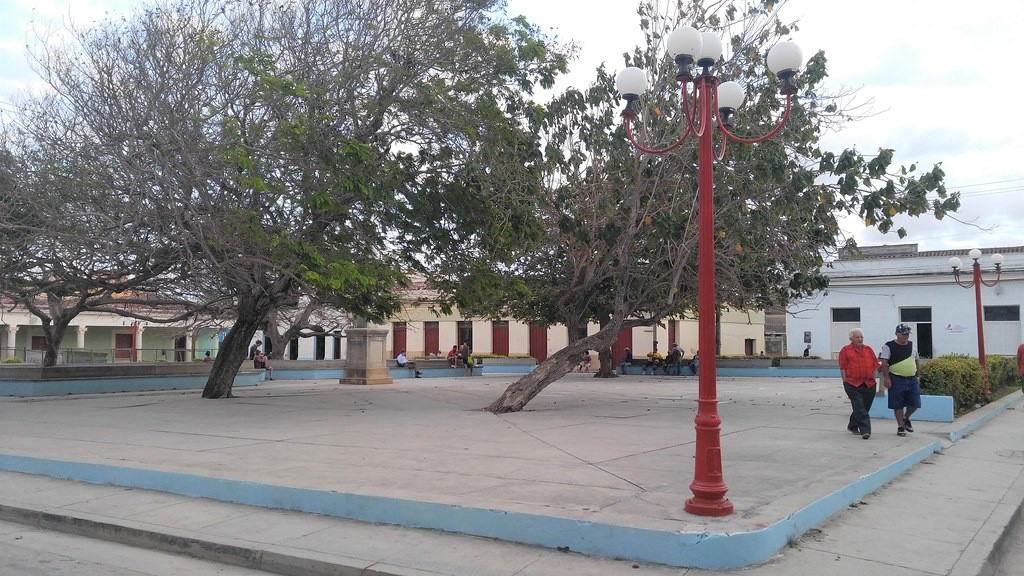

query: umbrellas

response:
[470,363,474,376]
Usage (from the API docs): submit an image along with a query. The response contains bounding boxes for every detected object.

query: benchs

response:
[0,359,538,397]
[619,357,883,378]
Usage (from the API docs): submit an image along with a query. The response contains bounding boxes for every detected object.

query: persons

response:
[1017,342,1024,394]
[838,329,879,439]
[397,348,422,378]
[878,346,884,374]
[250,340,274,380]
[666,343,684,376]
[760,350,765,358]
[428,350,442,357]
[804,345,811,357]
[204,351,215,363]
[447,340,471,376]
[688,350,699,376]
[881,324,921,436]
[642,348,663,376]
[576,350,591,373]
[620,347,632,374]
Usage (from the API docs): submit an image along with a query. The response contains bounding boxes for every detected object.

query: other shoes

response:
[415,375,421,378]
[848,423,862,435]
[862,433,870,439]
[650,370,654,375]
[415,371,423,375]
[451,365,456,369]
[642,370,646,374]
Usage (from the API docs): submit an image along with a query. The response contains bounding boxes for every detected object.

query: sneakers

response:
[902,417,914,432]
[897,427,907,437]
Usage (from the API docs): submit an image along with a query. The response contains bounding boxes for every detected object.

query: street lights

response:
[947,249,1005,398]
[615,25,802,518]
[121,317,148,362]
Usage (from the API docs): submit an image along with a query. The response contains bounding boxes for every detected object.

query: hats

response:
[896,324,912,335]
[624,347,630,350]
